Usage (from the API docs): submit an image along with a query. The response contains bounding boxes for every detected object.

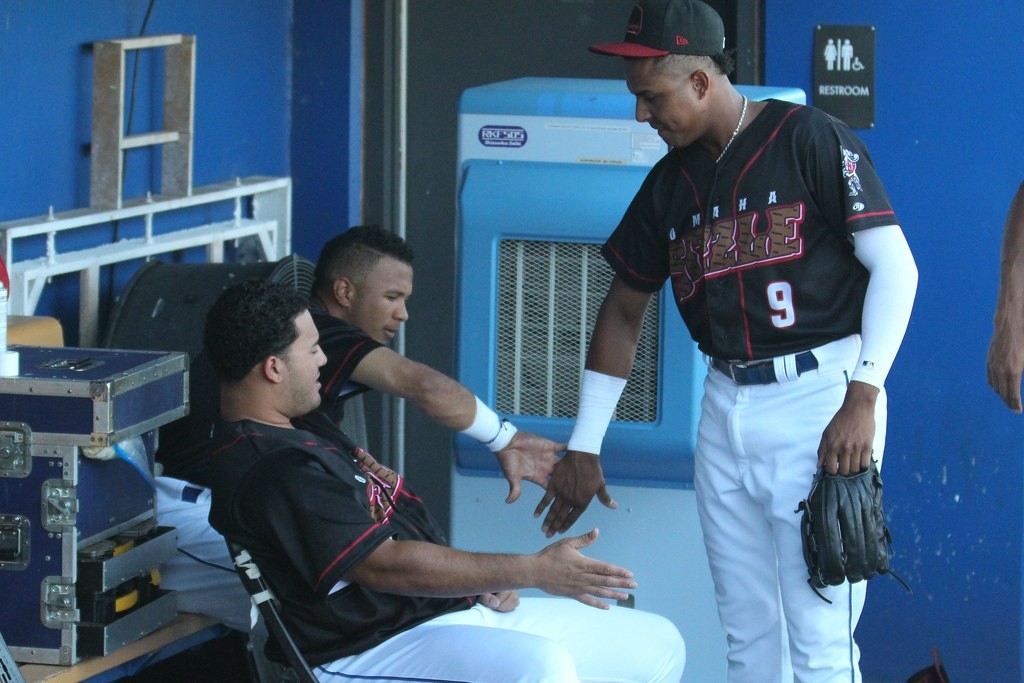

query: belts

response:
[182,486,203,502]
[713,352,818,385]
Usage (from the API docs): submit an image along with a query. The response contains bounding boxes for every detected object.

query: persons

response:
[986,180,1024,413]
[534,0,918,682]
[201,274,688,680]
[151,226,569,683]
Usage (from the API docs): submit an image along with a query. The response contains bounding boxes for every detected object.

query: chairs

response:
[227,538,319,683]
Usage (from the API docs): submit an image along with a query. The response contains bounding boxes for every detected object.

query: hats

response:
[589,0,726,57]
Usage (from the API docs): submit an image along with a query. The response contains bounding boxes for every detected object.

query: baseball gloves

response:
[800,455,889,588]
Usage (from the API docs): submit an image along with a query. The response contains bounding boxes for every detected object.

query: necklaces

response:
[716,95,748,163]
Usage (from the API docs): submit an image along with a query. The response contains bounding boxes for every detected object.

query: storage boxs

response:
[0,342,193,666]
